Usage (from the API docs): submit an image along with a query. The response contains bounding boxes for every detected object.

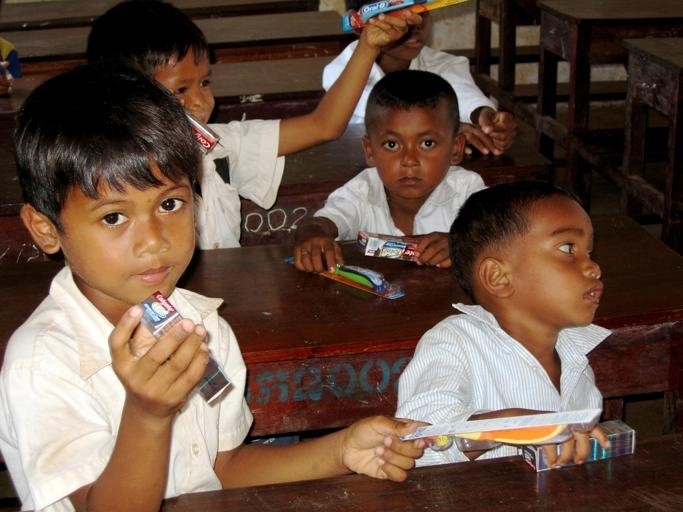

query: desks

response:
[0,1,683,512]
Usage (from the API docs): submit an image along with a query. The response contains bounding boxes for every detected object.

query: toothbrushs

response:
[285,253,407,302]
[400,409,603,454]
[336,0,467,31]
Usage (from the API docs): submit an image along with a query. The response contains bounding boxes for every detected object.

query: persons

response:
[1,61,431,512]
[0,37,20,96]
[86,2,434,253]
[392,181,614,473]
[288,69,490,272]
[319,1,518,162]
